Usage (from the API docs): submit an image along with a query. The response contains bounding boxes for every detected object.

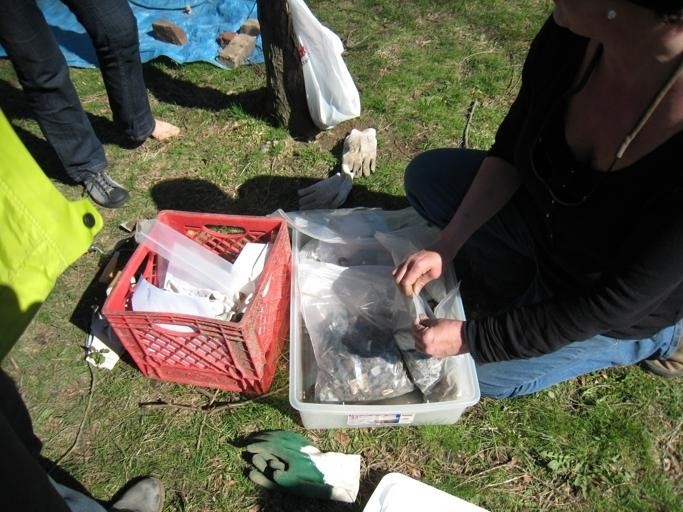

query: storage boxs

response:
[289,216,481,431]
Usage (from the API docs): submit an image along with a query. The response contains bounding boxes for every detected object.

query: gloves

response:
[243,429,361,503]
[341,128,376,176]
[296,173,352,211]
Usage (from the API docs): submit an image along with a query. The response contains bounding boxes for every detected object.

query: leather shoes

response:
[110,474,164,512]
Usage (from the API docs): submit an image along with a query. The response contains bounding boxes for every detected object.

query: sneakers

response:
[82,168,129,209]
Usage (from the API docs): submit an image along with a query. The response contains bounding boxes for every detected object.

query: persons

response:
[1,406,165,512]
[1,0,183,209]
[388,0,683,403]
